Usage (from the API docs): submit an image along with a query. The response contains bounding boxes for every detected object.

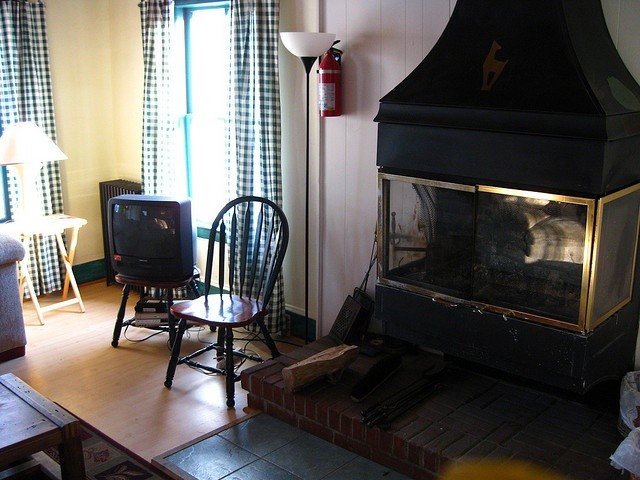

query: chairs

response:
[164,196,289,409]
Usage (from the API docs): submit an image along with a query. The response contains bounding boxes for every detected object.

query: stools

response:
[110,268,216,350]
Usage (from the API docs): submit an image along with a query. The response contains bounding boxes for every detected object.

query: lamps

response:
[279,31,338,347]
[1,121,68,222]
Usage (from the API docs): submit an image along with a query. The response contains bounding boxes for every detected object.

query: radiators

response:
[100,179,142,296]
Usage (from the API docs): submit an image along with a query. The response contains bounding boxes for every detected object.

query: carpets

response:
[0,401,175,480]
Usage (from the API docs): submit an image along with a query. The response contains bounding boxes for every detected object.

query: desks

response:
[0,213,88,326]
[0,372,88,479]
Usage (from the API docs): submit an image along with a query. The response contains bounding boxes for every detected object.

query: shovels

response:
[359,360,452,420]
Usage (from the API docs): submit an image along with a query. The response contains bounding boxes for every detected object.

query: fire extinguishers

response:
[318,39,343,117]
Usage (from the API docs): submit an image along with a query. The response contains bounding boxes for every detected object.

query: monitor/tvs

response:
[107,194,197,282]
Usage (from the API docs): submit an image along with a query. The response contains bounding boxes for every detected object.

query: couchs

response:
[0,236,28,364]
